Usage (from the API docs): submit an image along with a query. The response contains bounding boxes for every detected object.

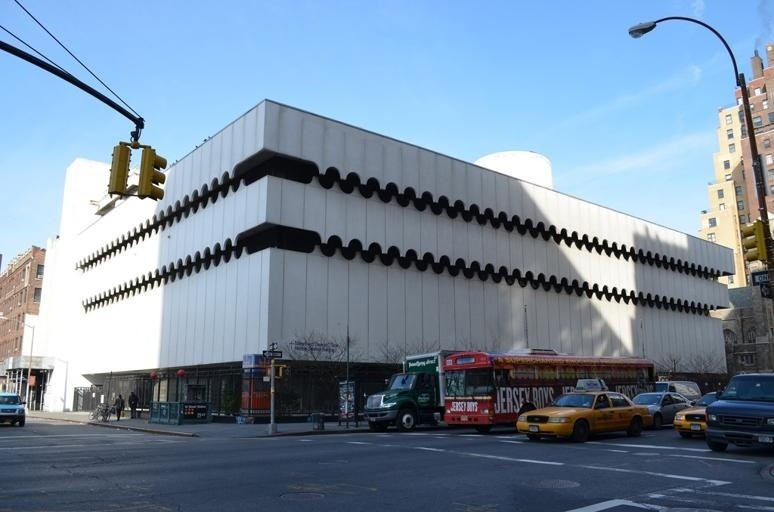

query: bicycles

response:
[89,401,115,423]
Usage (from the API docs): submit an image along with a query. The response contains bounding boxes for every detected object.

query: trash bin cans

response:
[311,413,325,430]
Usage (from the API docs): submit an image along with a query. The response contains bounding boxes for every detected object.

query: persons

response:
[129,392,139,419]
[113,394,125,421]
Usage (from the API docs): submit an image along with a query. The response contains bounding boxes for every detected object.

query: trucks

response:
[364,350,466,431]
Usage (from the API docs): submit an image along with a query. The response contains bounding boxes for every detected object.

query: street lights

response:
[0,317,35,412]
[630,18,770,269]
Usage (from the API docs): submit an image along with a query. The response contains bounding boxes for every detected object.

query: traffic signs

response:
[750,270,774,301]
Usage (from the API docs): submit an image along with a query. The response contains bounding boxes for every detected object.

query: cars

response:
[0,393,26,428]
[517,390,724,442]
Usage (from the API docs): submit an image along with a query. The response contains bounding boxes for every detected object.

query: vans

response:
[655,381,703,402]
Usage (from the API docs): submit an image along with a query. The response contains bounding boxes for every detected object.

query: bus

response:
[441,348,654,434]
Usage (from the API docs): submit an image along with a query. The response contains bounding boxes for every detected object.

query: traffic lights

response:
[107,146,167,202]
[738,219,768,265]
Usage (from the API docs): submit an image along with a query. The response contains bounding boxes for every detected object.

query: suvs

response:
[705,373,774,452]
